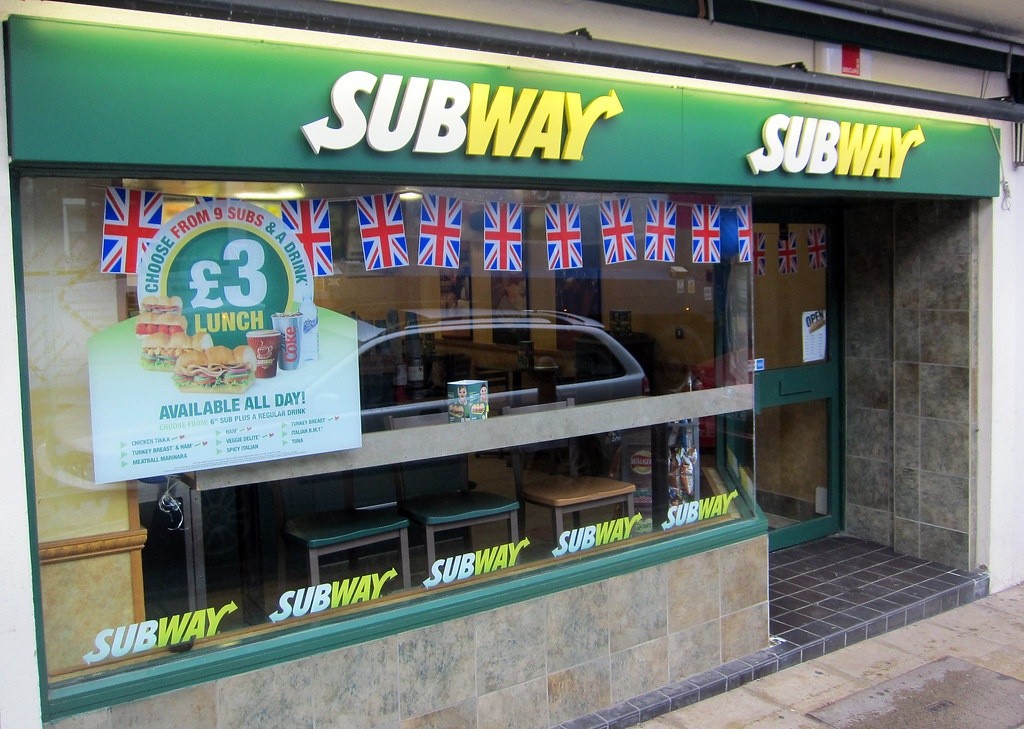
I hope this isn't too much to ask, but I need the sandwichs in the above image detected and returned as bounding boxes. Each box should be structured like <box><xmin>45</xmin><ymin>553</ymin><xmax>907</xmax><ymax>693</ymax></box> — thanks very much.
<box><xmin>134</xmin><ymin>296</ymin><xmax>256</xmax><ymax>393</ymax></box>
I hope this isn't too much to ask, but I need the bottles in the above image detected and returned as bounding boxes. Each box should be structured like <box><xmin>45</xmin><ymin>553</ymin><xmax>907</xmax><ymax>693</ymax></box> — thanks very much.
<box><xmin>298</xmin><ymin>294</ymin><xmax>320</xmax><ymax>363</ymax></box>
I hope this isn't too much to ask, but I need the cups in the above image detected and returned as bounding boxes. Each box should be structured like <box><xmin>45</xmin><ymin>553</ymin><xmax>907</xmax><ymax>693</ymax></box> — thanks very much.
<box><xmin>271</xmin><ymin>313</ymin><xmax>305</xmax><ymax>371</ymax></box>
<box><xmin>246</xmin><ymin>329</ymin><xmax>282</xmax><ymax>378</ymax></box>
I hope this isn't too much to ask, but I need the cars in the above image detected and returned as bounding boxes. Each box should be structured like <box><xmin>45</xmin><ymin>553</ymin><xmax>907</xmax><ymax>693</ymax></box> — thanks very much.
<box><xmin>691</xmin><ymin>345</ymin><xmax>752</xmax><ymax>454</ymax></box>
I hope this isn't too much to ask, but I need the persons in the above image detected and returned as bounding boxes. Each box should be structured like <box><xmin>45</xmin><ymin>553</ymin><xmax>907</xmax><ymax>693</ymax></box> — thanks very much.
<box><xmin>556</xmin><ymin>273</ymin><xmax>593</xmax><ymax>335</ymax></box>
<box><xmin>479</xmin><ymin>384</ymin><xmax>488</xmax><ymax>412</ymax></box>
<box><xmin>457</xmin><ymin>386</ymin><xmax>470</xmax><ymax>418</ymax></box>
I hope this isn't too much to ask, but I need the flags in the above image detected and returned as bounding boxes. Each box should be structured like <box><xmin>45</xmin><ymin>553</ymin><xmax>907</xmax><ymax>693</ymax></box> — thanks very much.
<box><xmin>777</xmin><ymin>232</ymin><xmax>798</xmax><ymax>276</ymax></box>
<box><xmin>545</xmin><ymin>202</ymin><xmax>583</xmax><ymax>270</ymax></box>
<box><xmin>753</xmin><ymin>232</ymin><xmax>766</xmax><ymax>275</ymax></box>
<box><xmin>281</xmin><ymin>197</ymin><xmax>333</xmax><ymax>277</ymax></box>
<box><xmin>691</xmin><ymin>204</ymin><xmax>721</xmax><ymax>263</ymax></box>
<box><xmin>417</xmin><ymin>192</ymin><xmax>463</xmax><ymax>269</ymax></box>
<box><xmin>194</xmin><ymin>196</ymin><xmax>242</xmax><ymax>205</ymax></box>
<box><xmin>644</xmin><ymin>198</ymin><xmax>677</xmax><ymax>263</ymax></box>
<box><xmin>736</xmin><ymin>205</ymin><xmax>752</xmax><ymax>262</ymax></box>
<box><xmin>807</xmin><ymin>228</ymin><xmax>827</xmax><ymax>270</ymax></box>
<box><xmin>99</xmin><ymin>185</ymin><xmax>165</xmax><ymax>273</ymax></box>
<box><xmin>483</xmin><ymin>201</ymin><xmax>523</xmax><ymax>271</ymax></box>
<box><xmin>599</xmin><ymin>198</ymin><xmax>637</xmax><ymax>265</ymax></box>
<box><xmin>356</xmin><ymin>192</ymin><xmax>410</xmax><ymax>272</ymax></box>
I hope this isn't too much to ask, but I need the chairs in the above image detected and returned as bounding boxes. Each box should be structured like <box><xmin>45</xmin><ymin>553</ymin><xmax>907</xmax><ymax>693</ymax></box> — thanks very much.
<box><xmin>501</xmin><ymin>397</ymin><xmax>636</xmax><ymax>553</ymax></box>
<box><xmin>384</xmin><ymin>412</ymin><xmax>520</xmax><ymax>580</ymax></box>
<box><xmin>277</xmin><ymin>461</ymin><xmax>410</xmax><ymax>596</ymax></box>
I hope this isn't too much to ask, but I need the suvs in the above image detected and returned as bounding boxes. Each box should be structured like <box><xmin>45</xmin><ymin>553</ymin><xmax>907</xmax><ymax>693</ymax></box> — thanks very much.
<box><xmin>142</xmin><ymin>309</ymin><xmax>657</xmax><ymax>549</ymax></box>
